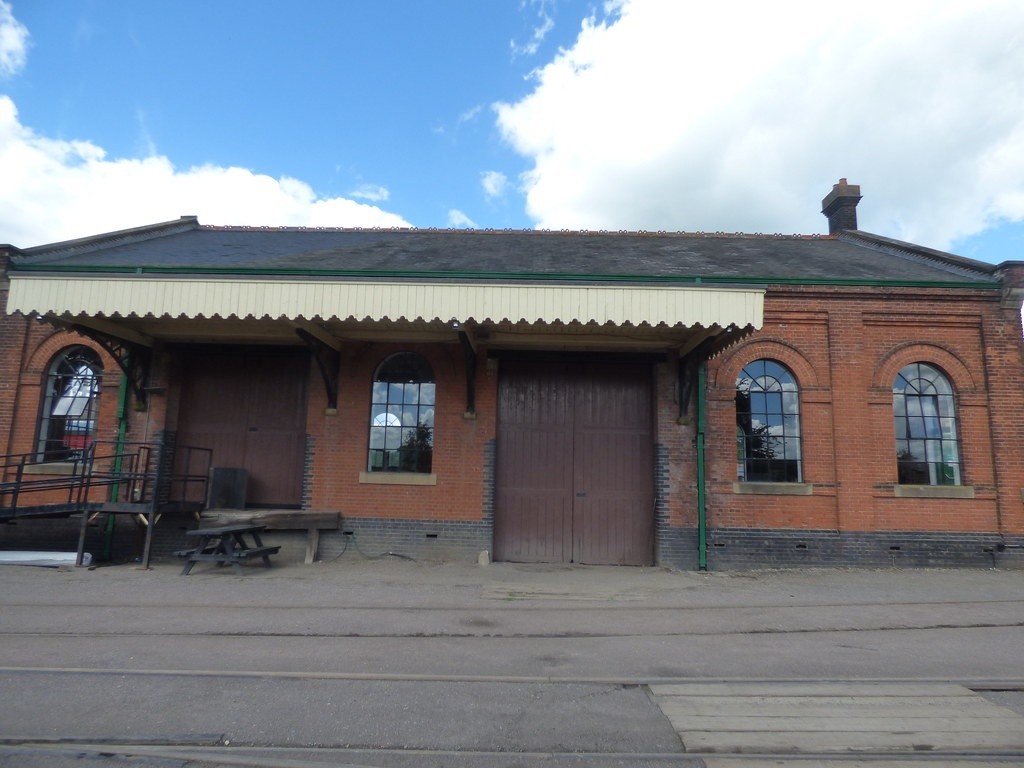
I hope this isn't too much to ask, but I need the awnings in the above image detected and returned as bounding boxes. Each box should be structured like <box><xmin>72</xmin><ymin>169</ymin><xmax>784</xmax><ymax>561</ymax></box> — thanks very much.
<box><xmin>5</xmin><ymin>266</ymin><xmax>764</xmax><ymax>572</ymax></box>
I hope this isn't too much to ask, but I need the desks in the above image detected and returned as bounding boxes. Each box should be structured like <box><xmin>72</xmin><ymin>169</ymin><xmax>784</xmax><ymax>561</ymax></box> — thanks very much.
<box><xmin>175</xmin><ymin>526</ymin><xmax>282</xmax><ymax>577</ymax></box>
